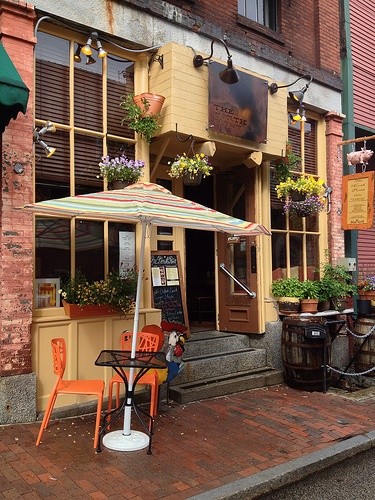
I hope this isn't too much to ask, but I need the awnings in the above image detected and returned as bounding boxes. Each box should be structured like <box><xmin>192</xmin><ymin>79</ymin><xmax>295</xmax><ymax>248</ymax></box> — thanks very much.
<box><xmin>0</xmin><ymin>42</ymin><xmax>30</xmax><ymax>134</ymax></box>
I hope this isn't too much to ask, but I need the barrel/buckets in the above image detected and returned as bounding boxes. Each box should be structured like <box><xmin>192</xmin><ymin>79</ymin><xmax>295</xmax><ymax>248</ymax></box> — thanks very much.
<box><xmin>280</xmin><ymin>317</ymin><xmax>331</xmax><ymax>391</ymax></box>
<box><xmin>353</xmin><ymin>317</ymin><xmax>375</xmax><ymax>378</ymax></box>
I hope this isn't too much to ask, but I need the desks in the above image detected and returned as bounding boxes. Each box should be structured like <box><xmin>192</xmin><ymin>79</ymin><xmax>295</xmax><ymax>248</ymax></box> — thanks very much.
<box><xmin>278</xmin><ymin>306</ymin><xmax>356</xmax><ymax>318</ymax></box>
<box><xmin>94</xmin><ymin>350</ymin><xmax>168</xmax><ymax>455</ymax></box>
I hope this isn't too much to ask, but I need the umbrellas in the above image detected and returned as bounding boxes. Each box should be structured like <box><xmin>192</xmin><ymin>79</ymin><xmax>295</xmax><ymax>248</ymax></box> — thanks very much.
<box><xmin>35</xmin><ymin>187</ymin><xmax>119</xmax><ymax>252</ymax></box>
<box><xmin>13</xmin><ymin>181</ymin><xmax>272</xmax><ymax>435</ymax></box>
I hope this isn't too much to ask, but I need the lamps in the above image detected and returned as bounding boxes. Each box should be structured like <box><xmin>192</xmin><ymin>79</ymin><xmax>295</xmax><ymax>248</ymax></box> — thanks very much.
<box><xmin>270</xmin><ymin>74</ymin><xmax>312</xmax><ymax>107</ymax></box>
<box><xmin>81</xmin><ymin>32</ymin><xmax>108</xmax><ymax>58</ymax></box>
<box><xmin>33</xmin><ymin>121</ymin><xmax>57</xmax><ymax>158</ymax></box>
<box><xmin>194</xmin><ymin>38</ymin><xmax>240</xmax><ymax>84</ymax></box>
<box><xmin>74</xmin><ymin>43</ymin><xmax>96</xmax><ymax>64</ymax></box>
<box><xmin>293</xmin><ymin>106</ymin><xmax>307</xmax><ymax>121</ymax></box>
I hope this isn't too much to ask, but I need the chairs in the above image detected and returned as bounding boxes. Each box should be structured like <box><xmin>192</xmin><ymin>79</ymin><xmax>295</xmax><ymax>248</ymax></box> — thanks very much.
<box><xmin>107</xmin><ymin>332</ymin><xmax>159</xmax><ymax>435</ymax></box>
<box><xmin>36</xmin><ymin>337</ymin><xmax>105</xmax><ymax>449</ymax></box>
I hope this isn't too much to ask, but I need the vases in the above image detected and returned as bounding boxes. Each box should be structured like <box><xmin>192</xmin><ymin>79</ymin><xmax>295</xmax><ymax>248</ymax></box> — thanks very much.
<box><xmin>289</xmin><ymin>189</ymin><xmax>305</xmax><ymax>201</ymax></box>
<box><xmin>62</xmin><ymin>298</ymin><xmax>133</xmax><ymax>318</ymax></box>
<box><xmin>111</xmin><ymin>179</ymin><xmax>133</xmax><ymax>190</ymax></box>
<box><xmin>184</xmin><ymin>168</ymin><xmax>201</xmax><ymax>186</ymax></box>
<box><xmin>359</xmin><ymin>289</ymin><xmax>375</xmax><ymax>300</ymax></box>
<box><xmin>296</xmin><ymin>207</ymin><xmax>310</xmax><ymax>217</ymax></box>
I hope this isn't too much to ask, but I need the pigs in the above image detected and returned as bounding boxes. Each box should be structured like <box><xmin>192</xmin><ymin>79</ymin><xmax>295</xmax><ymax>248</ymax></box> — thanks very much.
<box><xmin>347</xmin><ymin>150</ymin><xmax>374</xmax><ymax>166</ymax></box>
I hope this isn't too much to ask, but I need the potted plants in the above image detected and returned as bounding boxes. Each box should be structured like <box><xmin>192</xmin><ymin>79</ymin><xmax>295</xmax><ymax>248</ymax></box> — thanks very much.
<box><xmin>118</xmin><ymin>93</ymin><xmax>166</xmax><ymax>146</ymax></box>
<box><xmin>270</xmin><ymin>248</ymin><xmax>358</xmax><ymax>313</ymax></box>
<box><xmin>273</xmin><ymin>142</ymin><xmax>303</xmax><ymax>183</ymax></box>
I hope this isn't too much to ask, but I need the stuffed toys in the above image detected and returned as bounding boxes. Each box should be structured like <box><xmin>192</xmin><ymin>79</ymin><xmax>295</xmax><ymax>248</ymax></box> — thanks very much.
<box><xmin>165</xmin><ymin>332</ymin><xmax>185</xmax><ymax>382</ymax></box>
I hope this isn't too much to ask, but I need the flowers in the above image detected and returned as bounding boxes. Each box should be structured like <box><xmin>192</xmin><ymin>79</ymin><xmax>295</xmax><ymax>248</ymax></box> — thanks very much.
<box><xmin>95</xmin><ymin>150</ymin><xmax>145</xmax><ymax>184</ymax></box>
<box><xmin>58</xmin><ymin>261</ymin><xmax>149</xmax><ymax>321</ymax></box>
<box><xmin>362</xmin><ymin>275</ymin><xmax>375</xmax><ymax>291</ymax></box>
<box><xmin>274</xmin><ymin>175</ymin><xmax>324</xmax><ymax>222</ymax></box>
<box><xmin>166</xmin><ymin>152</ymin><xmax>213</xmax><ymax>179</ymax></box>
